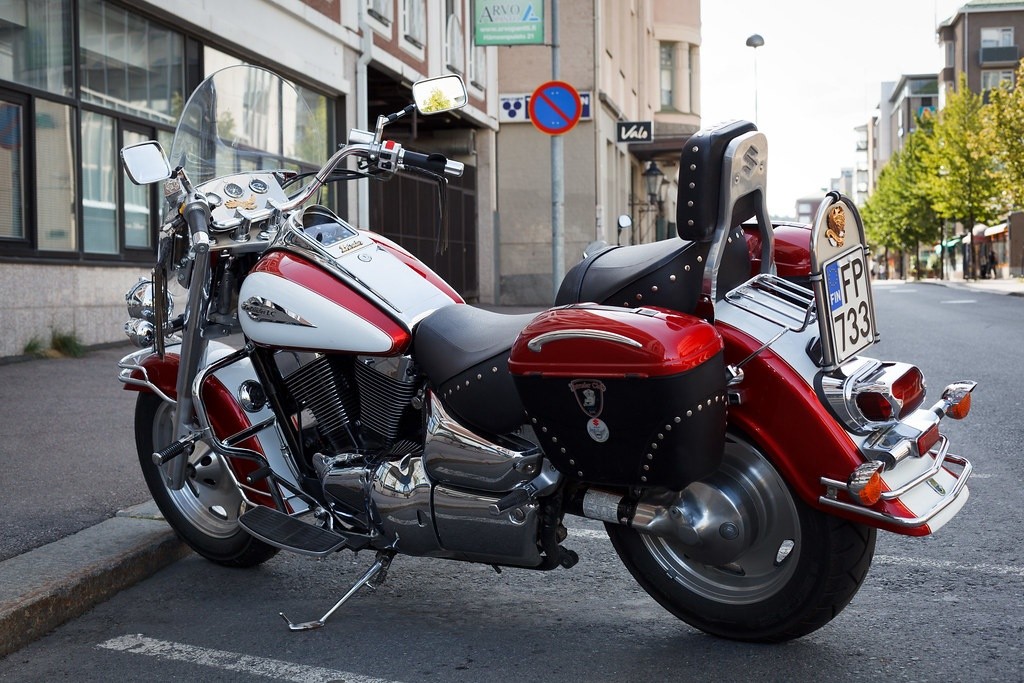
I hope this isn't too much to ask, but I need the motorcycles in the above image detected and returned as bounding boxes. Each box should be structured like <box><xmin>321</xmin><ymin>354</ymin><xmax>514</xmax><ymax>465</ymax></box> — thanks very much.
<box><xmin>116</xmin><ymin>64</ymin><xmax>980</xmax><ymax>645</ymax></box>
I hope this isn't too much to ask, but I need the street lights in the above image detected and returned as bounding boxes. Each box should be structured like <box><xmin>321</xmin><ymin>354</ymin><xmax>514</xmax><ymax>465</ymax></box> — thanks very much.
<box><xmin>746</xmin><ymin>33</ymin><xmax>765</xmax><ymax>126</ymax></box>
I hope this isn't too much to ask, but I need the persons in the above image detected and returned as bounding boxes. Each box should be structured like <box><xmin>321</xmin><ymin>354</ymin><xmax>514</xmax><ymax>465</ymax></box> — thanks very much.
<box><xmin>871</xmin><ymin>258</ymin><xmax>886</xmax><ymax>280</ymax></box>
<box><xmin>978</xmin><ymin>245</ymin><xmax>998</xmax><ymax>279</ymax></box>
